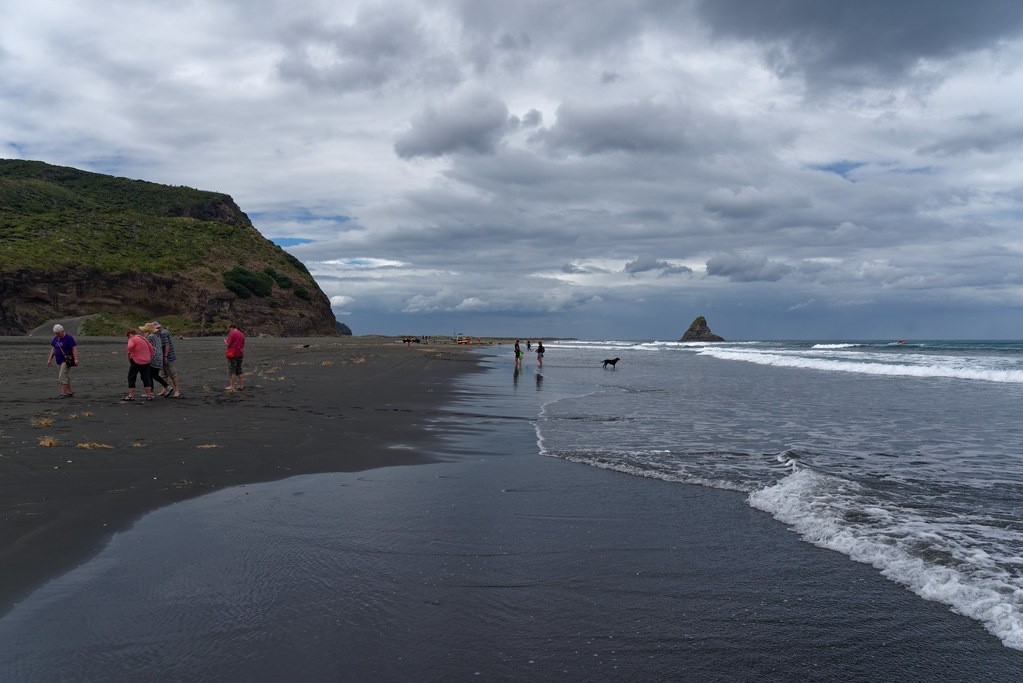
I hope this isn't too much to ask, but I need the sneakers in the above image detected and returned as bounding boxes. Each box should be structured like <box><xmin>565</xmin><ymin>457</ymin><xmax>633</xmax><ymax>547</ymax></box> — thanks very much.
<box><xmin>164</xmin><ymin>387</ymin><xmax>173</xmax><ymax>397</ymax></box>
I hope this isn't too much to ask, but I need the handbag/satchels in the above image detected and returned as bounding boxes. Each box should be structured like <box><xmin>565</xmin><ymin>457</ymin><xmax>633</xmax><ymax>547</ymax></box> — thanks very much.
<box><xmin>65</xmin><ymin>354</ymin><xmax>78</xmax><ymax>367</ymax></box>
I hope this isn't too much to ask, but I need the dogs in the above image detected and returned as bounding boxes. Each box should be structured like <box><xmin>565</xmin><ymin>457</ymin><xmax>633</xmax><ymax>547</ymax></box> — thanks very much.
<box><xmin>600</xmin><ymin>358</ymin><xmax>620</xmax><ymax>369</ymax></box>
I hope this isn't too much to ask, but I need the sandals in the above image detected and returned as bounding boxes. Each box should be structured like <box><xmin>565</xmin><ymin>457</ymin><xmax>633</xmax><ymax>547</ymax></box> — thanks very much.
<box><xmin>120</xmin><ymin>396</ymin><xmax>135</xmax><ymax>401</ymax></box>
<box><xmin>146</xmin><ymin>396</ymin><xmax>152</xmax><ymax>401</ymax></box>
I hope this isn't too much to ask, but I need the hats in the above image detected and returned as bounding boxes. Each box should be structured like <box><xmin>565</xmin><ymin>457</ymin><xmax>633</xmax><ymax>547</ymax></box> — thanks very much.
<box><xmin>139</xmin><ymin>323</ymin><xmax>159</xmax><ymax>333</ymax></box>
<box><xmin>151</xmin><ymin>322</ymin><xmax>162</xmax><ymax>328</ymax></box>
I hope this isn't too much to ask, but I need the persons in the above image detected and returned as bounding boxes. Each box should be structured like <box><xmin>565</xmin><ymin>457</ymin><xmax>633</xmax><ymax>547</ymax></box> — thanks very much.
<box><xmin>408</xmin><ymin>338</ymin><xmax>414</xmax><ymax>351</ymax></box>
<box><xmin>526</xmin><ymin>340</ymin><xmax>532</xmax><ymax>351</ymax></box>
<box><xmin>137</xmin><ymin>322</ymin><xmax>174</xmax><ymax>398</ymax></box>
<box><xmin>514</xmin><ymin>340</ymin><xmax>521</xmax><ymax>364</ymax></box>
<box><xmin>152</xmin><ymin>321</ymin><xmax>181</xmax><ymax>398</ymax></box>
<box><xmin>535</xmin><ymin>340</ymin><xmax>545</xmax><ymax>368</ymax></box>
<box><xmin>46</xmin><ymin>324</ymin><xmax>79</xmax><ymax>399</ymax></box>
<box><xmin>519</xmin><ymin>351</ymin><xmax>523</xmax><ymax>364</ymax></box>
<box><xmin>223</xmin><ymin>323</ymin><xmax>245</xmax><ymax>390</ymax></box>
<box><xmin>121</xmin><ymin>327</ymin><xmax>155</xmax><ymax>401</ymax></box>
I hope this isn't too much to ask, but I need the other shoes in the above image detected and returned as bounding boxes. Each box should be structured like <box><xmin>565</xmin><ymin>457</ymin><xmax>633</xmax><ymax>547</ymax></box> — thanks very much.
<box><xmin>56</xmin><ymin>393</ymin><xmax>74</xmax><ymax>399</ymax></box>
